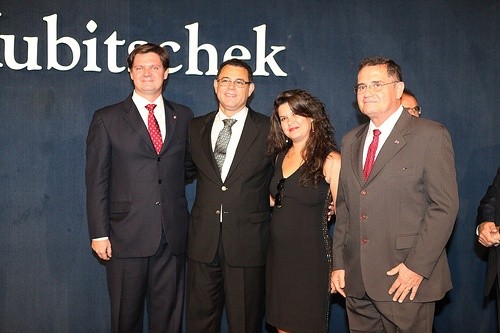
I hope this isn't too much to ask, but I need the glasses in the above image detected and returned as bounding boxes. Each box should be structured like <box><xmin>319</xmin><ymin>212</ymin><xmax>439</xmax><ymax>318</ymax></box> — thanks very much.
<box><xmin>274</xmin><ymin>177</ymin><xmax>286</xmax><ymax>208</ymax></box>
<box><xmin>404</xmin><ymin>105</ymin><xmax>422</xmax><ymax>115</ymax></box>
<box><xmin>353</xmin><ymin>80</ymin><xmax>398</xmax><ymax>94</ymax></box>
<box><xmin>216</xmin><ymin>77</ymin><xmax>252</xmax><ymax>87</ymax></box>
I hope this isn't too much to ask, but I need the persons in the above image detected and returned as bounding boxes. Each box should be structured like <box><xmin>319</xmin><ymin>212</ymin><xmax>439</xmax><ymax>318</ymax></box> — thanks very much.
<box><xmin>182</xmin><ymin>59</ymin><xmax>334</xmax><ymax>333</ymax></box>
<box><xmin>401</xmin><ymin>90</ymin><xmax>421</xmax><ymax>118</ymax></box>
<box><xmin>330</xmin><ymin>56</ymin><xmax>460</xmax><ymax>333</ymax></box>
<box><xmin>475</xmin><ymin>165</ymin><xmax>500</xmax><ymax>322</ymax></box>
<box><xmin>84</xmin><ymin>42</ymin><xmax>195</xmax><ymax>333</ymax></box>
<box><xmin>263</xmin><ymin>90</ymin><xmax>341</xmax><ymax>333</ymax></box>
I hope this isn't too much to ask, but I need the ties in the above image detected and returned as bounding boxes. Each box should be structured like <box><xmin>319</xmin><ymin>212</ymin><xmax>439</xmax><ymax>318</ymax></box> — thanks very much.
<box><xmin>145</xmin><ymin>104</ymin><xmax>163</xmax><ymax>154</ymax></box>
<box><xmin>213</xmin><ymin>119</ymin><xmax>238</xmax><ymax>177</ymax></box>
<box><xmin>362</xmin><ymin>127</ymin><xmax>383</xmax><ymax>184</ymax></box>
